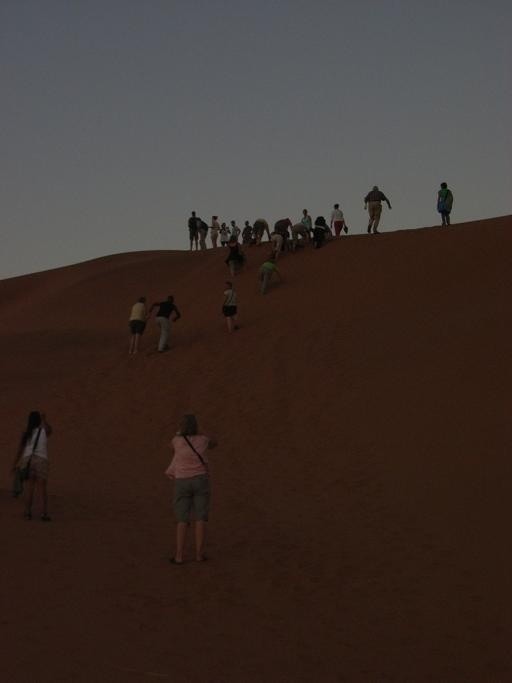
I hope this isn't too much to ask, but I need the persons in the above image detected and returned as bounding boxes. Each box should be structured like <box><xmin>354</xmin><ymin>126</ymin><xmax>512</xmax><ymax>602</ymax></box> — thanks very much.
<box><xmin>363</xmin><ymin>186</ymin><xmax>391</xmax><ymax>235</ymax></box>
<box><xmin>166</xmin><ymin>409</ymin><xmax>218</xmax><ymax>565</ymax></box>
<box><xmin>437</xmin><ymin>182</ymin><xmax>454</xmax><ymax>228</ymax></box>
<box><xmin>225</xmin><ymin>235</ymin><xmax>244</xmax><ymax>275</ymax></box>
<box><xmin>258</xmin><ymin>256</ymin><xmax>281</xmax><ymax>294</ymax></box>
<box><xmin>222</xmin><ymin>281</ymin><xmax>238</xmax><ymax>334</ymax></box>
<box><xmin>6</xmin><ymin>409</ymin><xmax>52</xmax><ymax>522</ymax></box>
<box><xmin>128</xmin><ymin>295</ymin><xmax>180</xmax><ymax>352</ymax></box>
<box><xmin>188</xmin><ymin>204</ymin><xmax>346</xmax><ymax>260</ymax></box>
